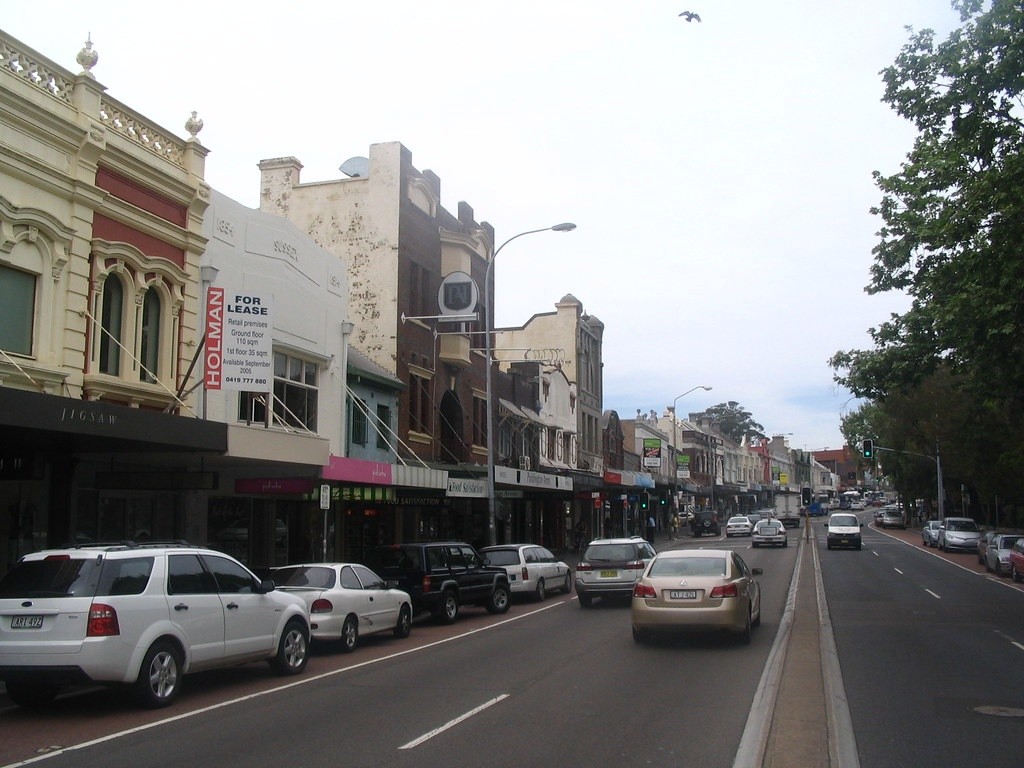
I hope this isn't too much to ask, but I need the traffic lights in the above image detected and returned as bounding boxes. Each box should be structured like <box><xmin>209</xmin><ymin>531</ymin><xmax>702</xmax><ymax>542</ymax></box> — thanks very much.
<box><xmin>640</xmin><ymin>492</ymin><xmax>649</xmax><ymax>512</ymax></box>
<box><xmin>802</xmin><ymin>488</ymin><xmax>811</xmax><ymax>506</ymax></box>
<box><xmin>659</xmin><ymin>491</ymin><xmax>667</xmax><ymax>507</ymax></box>
<box><xmin>862</xmin><ymin>440</ymin><xmax>873</xmax><ymax>458</ymax></box>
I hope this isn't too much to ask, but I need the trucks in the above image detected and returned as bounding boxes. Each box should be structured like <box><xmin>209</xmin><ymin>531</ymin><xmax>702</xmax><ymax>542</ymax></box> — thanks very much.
<box><xmin>773</xmin><ymin>492</ymin><xmax>801</xmax><ymax>528</ymax></box>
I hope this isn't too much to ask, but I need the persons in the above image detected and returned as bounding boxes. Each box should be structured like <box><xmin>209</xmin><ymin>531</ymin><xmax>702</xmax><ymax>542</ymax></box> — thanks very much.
<box><xmin>575</xmin><ymin>516</ymin><xmax>589</xmax><ymax>551</ymax></box>
<box><xmin>672</xmin><ymin>513</ymin><xmax>680</xmax><ymax>540</ymax></box>
<box><xmin>917</xmin><ymin>507</ymin><xmax>922</xmax><ymax>522</ymax></box>
<box><xmin>647</xmin><ymin>513</ymin><xmax>656</xmax><ymax>547</ymax></box>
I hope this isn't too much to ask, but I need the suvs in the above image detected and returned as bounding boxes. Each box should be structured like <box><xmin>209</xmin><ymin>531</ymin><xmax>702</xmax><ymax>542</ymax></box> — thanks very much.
<box><xmin>364</xmin><ymin>541</ymin><xmax>513</xmax><ymax>624</ymax></box>
<box><xmin>574</xmin><ymin>535</ymin><xmax>658</xmax><ymax>607</ymax></box>
<box><xmin>0</xmin><ymin>540</ymin><xmax>313</xmax><ymax>711</ymax></box>
<box><xmin>694</xmin><ymin>511</ymin><xmax>722</xmax><ymax>537</ymax></box>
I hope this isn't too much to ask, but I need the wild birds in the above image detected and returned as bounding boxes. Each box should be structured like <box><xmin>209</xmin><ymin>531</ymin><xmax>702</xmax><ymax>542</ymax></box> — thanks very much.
<box><xmin>679</xmin><ymin>11</ymin><xmax>702</xmax><ymax>22</ymax></box>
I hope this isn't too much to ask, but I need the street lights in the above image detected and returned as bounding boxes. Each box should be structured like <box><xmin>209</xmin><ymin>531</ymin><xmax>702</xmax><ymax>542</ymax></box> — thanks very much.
<box><xmin>673</xmin><ymin>385</ymin><xmax>712</xmax><ymax>517</ymax></box>
<box><xmin>485</xmin><ymin>222</ymin><xmax>577</xmax><ymax>544</ymax></box>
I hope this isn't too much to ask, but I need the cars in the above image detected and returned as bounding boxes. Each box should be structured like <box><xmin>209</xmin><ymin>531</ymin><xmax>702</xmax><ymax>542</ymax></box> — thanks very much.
<box><xmin>751</xmin><ymin>519</ymin><xmax>787</xmax><ymax>547</ymax></box>
<box><xmin>269</xmin><ymin>563</ymin><xmax>415</xmax><ymax>654</ymax></box>
<box><xmin>801</xmin><ymin>490</ymin><xmax>924</xmax><ymax>529</ymax></box>
<box><xmin>824</xmin><ymin>513</ymin><xmax>864</xmax><ymax>551</ymax></box>
<box><xmin>676</xmin><ymin>512</ymin><xmax>693</xmax><ymax>527</ymax></box>
<box><xmin>922</xmin><ymin>517</ymin><xmax>1024</xmax><ymax>583</ymax></box>
<box><xmin>482</xmin><ymin>543</ymin><xmax>573</xmax><ymax>603</ymax></box>
<box><xmin>726</xmin><ymin>509</ymin><xmax>775</xmax><ymax>538</ymax></box>
<box><xmin>630</xmin><ymin>549</ymin><xmax>764</xmax><ymax>645</ymax></box>
